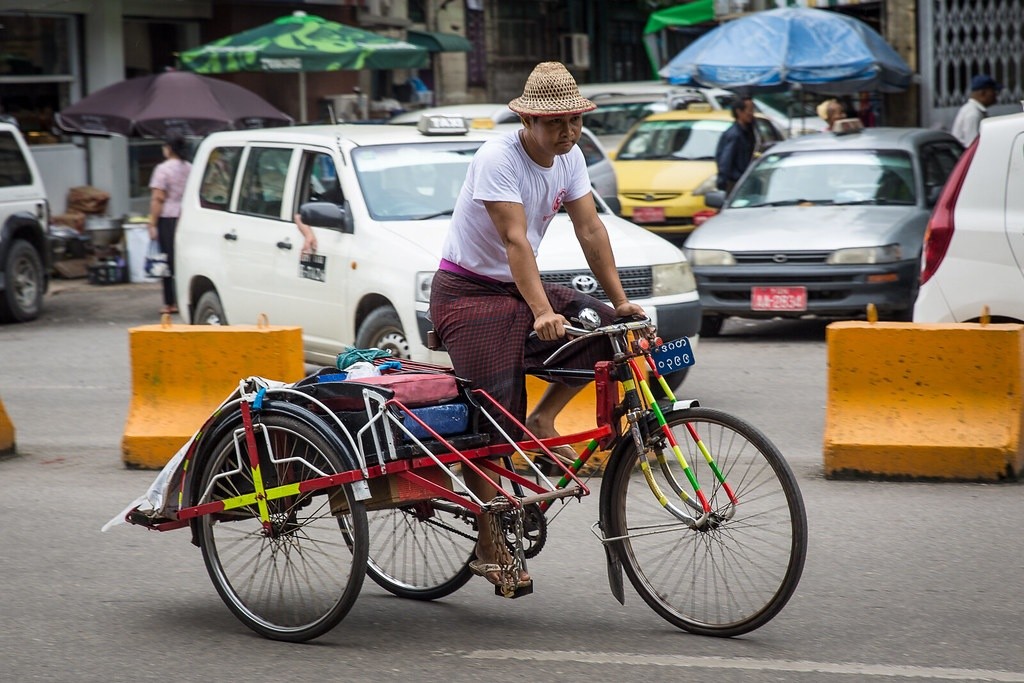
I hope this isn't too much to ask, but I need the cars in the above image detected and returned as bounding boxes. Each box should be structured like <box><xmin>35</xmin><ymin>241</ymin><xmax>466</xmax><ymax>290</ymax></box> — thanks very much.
<box><xmin>673</xmin><ymin>122</ymin><xmax>973</xmax><ymax>339</ymax></box>
<box><xmin>0</xmin><ymin>117</ymin><xmax>55</xmax><ymax>322</ymax></box>
<box><xmin>386</xmin><ymin>84</ymin><xmax>699</xmax><ymax>218</ymax></box>
<box><xmin>910</xmin><ymin>104</ymin><xmax>1024</xmax><ymax>335</ymax></box>
<box><xmin>610</xmin><ymin>101</ymin><xmax>786</xmax><ymax>248</ymax></box>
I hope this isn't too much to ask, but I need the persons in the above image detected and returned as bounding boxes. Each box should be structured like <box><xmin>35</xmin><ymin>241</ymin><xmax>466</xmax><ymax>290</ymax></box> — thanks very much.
<box><xmin>817</xmin><ymin>99</ymin><xmax>846</xmax><ymax>132</ymax></box>
<box><xmin>716</xmin><ymin>94</ymin><xmax>764</xmax><ymax>201</ymax></box>
<box><xmin>148</xmin><ymin>135</ymin><xmax>194</xmax><ymax>314</ymax></box>
<box><xmin>293</xmin><ymin>205</ymin><xmax>345</xmax><ymax>254</ymax></box>
<box><xmin>951</xmin><ymin>74</ymin><xmax>1008</xmax><ymax>149</ymax></box>
<box><xmin>429</xmin><ymin>61</ymin><xmax>648</xmax><ymax>585</ymax></box>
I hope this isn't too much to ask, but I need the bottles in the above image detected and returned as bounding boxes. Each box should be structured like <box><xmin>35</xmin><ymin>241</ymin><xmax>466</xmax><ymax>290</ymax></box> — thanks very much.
<box><xmin>97</xmin><ymin>257</ymin><xmax>117</xmax><ymax>280</ymax></box>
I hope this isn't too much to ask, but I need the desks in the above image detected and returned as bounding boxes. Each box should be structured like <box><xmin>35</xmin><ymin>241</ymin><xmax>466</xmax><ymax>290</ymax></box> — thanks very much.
<box><xmin>87</xmin><ymin>226</ymin><xmax>123</xmax><ymax>244</ymax></box>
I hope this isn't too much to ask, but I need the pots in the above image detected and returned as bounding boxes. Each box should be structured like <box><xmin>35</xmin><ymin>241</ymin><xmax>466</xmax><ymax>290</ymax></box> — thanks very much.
<box><xmin>85</xmin><ymin>218</ymin><xmax>123</xmax><ymax>244</ymax></box>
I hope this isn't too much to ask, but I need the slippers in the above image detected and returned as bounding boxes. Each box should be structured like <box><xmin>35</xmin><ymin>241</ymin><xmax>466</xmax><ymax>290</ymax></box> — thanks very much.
<box><xmin>159</xmin><ymin>306</ymin><xmax>179</xmax><ymax>313</ymax></box>
<box><xmin>469</xmin><ymin>558</ymin><xmax>531</xmax><ymax>587</ymax></box>
<box><xmin>525</xmin><ymin>445</ymin><xmax>589</xmax><ymax>468</ymax></box>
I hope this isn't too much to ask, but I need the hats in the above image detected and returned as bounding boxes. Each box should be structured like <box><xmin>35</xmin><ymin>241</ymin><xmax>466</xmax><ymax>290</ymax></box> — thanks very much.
<box><xmin>508</xmin><ymin>62</ymin><xmax>597</xmax><ymax>116</ymax></box>
<box><xmin>972</xmin><ymin>76</ymin><xmax>1004</xmax><ymax>90</ymax></box>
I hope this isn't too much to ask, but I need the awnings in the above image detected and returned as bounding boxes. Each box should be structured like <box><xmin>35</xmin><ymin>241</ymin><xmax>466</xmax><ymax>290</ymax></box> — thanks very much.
<box><xmin>406</xmin><ymin>29</ymin><xmax>472</xmax><ymax>52</ymax></box>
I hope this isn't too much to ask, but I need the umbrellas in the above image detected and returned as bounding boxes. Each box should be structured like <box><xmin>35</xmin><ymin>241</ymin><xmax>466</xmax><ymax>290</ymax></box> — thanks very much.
<box><xmin>50</xmin><ymin>68</ymin><xmax>294</xmax><ymax>141</ymax></box>
<box><xmin>657</xmin><ymin>4</ymin><xmax>914</xmax><ymax>90</ymax></box>
<box><xmin>173</xmin><ymin>11</ymin><xmax>431</xmax><ymax>124</ymax></box>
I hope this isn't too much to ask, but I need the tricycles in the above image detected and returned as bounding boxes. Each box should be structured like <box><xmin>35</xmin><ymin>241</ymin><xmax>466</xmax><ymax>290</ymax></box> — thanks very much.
<box><xmin>122</xmin><ymin>308</ymin><xmax>810</xmax><ymax>648</ymax></box>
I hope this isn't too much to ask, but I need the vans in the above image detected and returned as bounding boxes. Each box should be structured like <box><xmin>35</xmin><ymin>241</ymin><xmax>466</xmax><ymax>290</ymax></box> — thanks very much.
<box><xmin>169</xmin><ymin>115</ymin><xmax>703</xmax><ymax>396</ymax></box>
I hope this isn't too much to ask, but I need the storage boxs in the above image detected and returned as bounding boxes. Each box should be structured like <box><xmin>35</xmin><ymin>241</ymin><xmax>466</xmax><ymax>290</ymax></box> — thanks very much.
<box><xmin>48</xmin><ymin>236</ymin><xmax>81</xmax><ymax>262</ymax></box>
<box><xmin>84</xmin><ymin>262</ymin><xmax>128</xmax><ymax>286</ymax></box>
<box><xmin>66</xmin><ymin>185</ymin><xmax>109</xmax><ymax>212</ymax></box>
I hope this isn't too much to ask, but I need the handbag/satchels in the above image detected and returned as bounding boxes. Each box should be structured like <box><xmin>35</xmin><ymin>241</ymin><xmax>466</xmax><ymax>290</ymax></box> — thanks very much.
<box><xmin>146</xmin><ymin>241</ymin><xmax>172</xmax><ymax>278</ymax></box>
